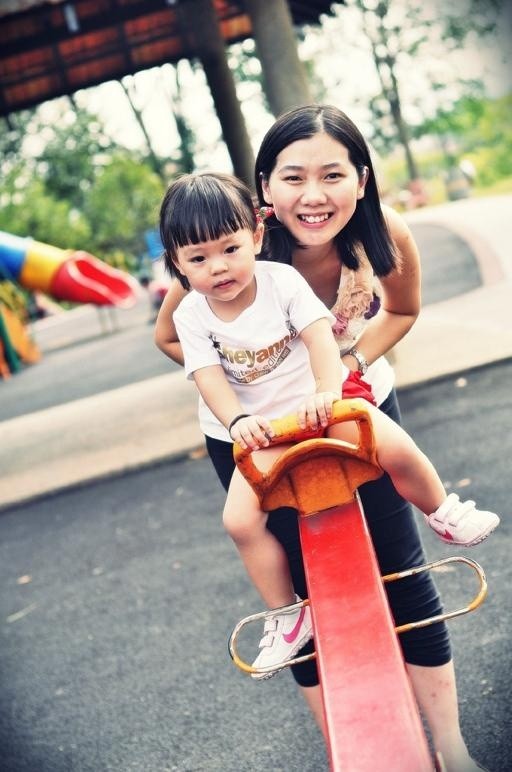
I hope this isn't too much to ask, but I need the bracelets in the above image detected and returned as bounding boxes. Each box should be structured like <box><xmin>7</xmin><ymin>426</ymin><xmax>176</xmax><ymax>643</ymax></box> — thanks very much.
<box><xmin>229</xmin><ymin>414</ymin><xmax>250</xmax><ymax>432</ymax></box>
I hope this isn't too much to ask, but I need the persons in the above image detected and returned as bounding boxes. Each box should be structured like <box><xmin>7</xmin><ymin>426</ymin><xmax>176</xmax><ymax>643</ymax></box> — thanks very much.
<box><xmin>154</xmin><ymin>106</ymin><xmax>486</xmax><ymax>772</ymax></box>
<box><xmin>406</xmin><ymin>170</ymin><xmax>424</xmax><ymax>209</ymax></box>
<box><xmin>159</xmin><ymin>173</ymin><xmax>500</xmax><ymax>681</ymax></box>
<box><xmin>444</xmin><ymin>162</ymin><xmax>470</xmax><ymax>198</ymax></box>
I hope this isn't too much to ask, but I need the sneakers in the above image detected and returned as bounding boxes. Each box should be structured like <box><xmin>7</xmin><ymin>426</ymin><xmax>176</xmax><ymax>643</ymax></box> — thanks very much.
<box><xmin>423</xmin><ymin>493</ymin><xmax>501</xmax><ymax>548</ymax></box>
<box><xmin>250</xmin><ymin>594</ymin><xmax>313</xmax><ymax>682</ymax></box>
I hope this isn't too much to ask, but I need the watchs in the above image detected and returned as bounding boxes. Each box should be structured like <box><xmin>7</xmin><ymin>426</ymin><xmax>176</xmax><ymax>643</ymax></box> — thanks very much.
<box><xmin>340</xmin><ymin>350</ymin><xmax>368</xmax><ymax>378</ymax></box>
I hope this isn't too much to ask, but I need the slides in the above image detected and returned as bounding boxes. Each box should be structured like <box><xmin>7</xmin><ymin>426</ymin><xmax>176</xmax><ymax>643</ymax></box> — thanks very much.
<box><xmin>0</xmin><ymin>231</ymin><xmax>141</xmax><ymax>306</ymax></box>
